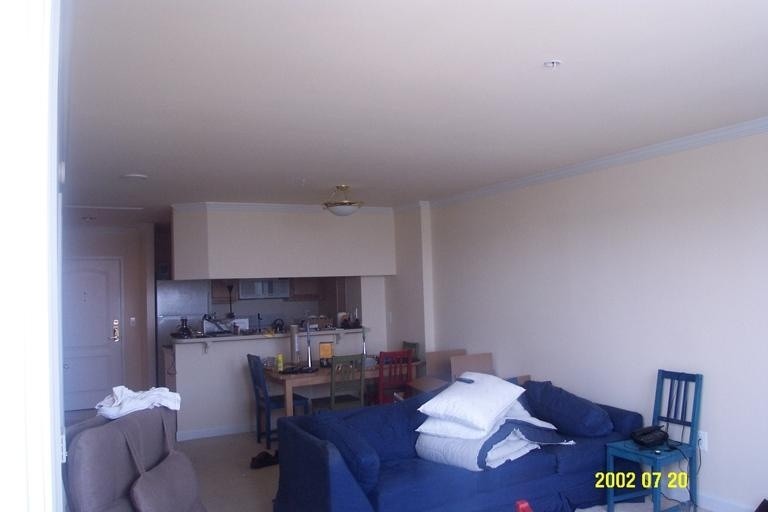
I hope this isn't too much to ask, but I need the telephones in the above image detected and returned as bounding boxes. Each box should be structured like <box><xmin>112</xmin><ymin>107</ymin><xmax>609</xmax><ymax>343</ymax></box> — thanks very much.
<box><xmin>630</xmin><ymin>424</ymin><xmax>668</xmax><ymax>447</ymax></box>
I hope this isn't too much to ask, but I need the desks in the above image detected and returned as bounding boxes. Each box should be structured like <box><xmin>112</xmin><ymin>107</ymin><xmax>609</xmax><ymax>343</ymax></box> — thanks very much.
<box><xmin>266</xmin><ymin>354</ymin><xmax>424</xmax><ymax>416</ymax></box>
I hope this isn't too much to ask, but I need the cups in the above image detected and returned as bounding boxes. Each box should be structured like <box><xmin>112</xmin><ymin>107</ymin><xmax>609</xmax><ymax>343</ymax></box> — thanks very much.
<box><xmin>267</xmin><ymin>357</ymin><xmax>275</xmax><ymax>366</ymax></box>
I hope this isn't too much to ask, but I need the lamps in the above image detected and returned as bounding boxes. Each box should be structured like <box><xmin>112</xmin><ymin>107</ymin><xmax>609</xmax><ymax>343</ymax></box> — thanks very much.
<box><xmin>321</xmin><ymin>184</ymin><xmax>364</xmax><ymax>216</ymax></box>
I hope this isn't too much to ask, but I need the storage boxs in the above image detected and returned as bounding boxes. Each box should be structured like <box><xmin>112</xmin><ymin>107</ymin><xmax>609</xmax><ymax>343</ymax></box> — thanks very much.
<box><xmin>407</xmin><ymin>350</ymin><xmax>531</xmax><ymax>395</ymax></box>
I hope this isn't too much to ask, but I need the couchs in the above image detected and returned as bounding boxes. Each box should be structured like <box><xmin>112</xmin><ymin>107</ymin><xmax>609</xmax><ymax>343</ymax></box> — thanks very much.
<box><xmin>272</xmin><ymin>380</ymin><xmax>647</xmax><ymax>512</ymax></box>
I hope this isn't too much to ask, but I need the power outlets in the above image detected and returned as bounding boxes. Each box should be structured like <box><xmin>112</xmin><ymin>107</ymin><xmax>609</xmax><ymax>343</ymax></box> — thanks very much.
<box><xmin>695</xmin><ymin>431</ymin><xmax>707</xmax><ymax>451</ymax></box>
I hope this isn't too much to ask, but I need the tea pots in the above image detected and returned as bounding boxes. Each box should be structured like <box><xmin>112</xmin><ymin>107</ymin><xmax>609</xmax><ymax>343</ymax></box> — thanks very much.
<box><xmin>272</xmin><ymin>319</ymin><xmax>287</xmax><ymax>334</ymax></box>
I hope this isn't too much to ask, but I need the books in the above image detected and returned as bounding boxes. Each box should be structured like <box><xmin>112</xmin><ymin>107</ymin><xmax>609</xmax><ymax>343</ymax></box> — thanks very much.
<box><xmin>279</xmin><ymin>367</ymin><xmax>319</xmax><ymax>373</ymax></box>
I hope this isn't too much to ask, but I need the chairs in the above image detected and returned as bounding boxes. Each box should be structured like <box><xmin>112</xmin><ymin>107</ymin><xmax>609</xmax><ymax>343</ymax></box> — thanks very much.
<box><xmin>402</xmin><ymin>340</ymin><xmax>420</xmax><ymax>360</ymax></box>
<box><xmin>606</xmin><ymin>368</ymin><xmax>703</xmax><ymax>512</ymax></box>
<box><xmin>311</xmin><ymin>352</ymin><xmax>365</xmax><ymax>414</ymax></box>
<box><xmin>247</xmin><ymin>354</ymin><xmax>310</xmax><ymax>450</ymax></box>
<box><xmin>366</xmin><ymin>349</ymin><xmax>412</xmax><ymax>405</ymax></box>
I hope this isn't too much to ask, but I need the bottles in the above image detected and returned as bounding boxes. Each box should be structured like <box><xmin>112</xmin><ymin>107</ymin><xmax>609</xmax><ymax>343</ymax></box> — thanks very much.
<box><xmin>179</xmin><ymin>319</ymin><xmax>189</xmax><ymax>338</ymax></box>
<box><xmin>277</xmin><ymin>353</ymin><xmax>283</xmax><ymax>372</ymax></box>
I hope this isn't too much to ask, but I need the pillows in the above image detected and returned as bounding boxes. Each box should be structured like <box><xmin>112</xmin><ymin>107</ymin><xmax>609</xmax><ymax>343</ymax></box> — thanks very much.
<box><xmin>414</xmin><ymin>400</ymin><xmax>516</xmax><ymax>440</ymax></box>
<box><xmin>417</xmin><ymin>372</ymin><xmax>527</xmax><ymax>431</ymax></box>
